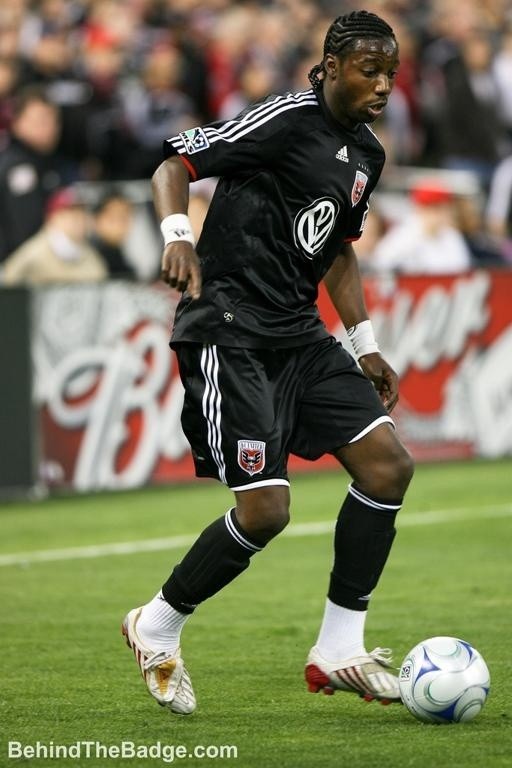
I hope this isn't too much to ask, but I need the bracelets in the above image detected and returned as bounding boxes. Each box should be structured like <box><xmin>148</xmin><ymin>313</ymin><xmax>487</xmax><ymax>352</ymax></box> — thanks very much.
<box><xmin>160</xmin><ymin>214</ymin><xmax>196</xmax><ymax>250</ymax></box>
<box><xmin>346</xmin><ymin>320</ymin><xmax>382</xmax><ymax>361</ymax></box>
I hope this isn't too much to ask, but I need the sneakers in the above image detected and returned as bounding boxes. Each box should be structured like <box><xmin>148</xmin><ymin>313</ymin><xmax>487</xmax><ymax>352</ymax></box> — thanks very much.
<box><xmin>305</xmin><ymin>645</ymin><xmax>400</xmax><ymax>704</ymax></box>
<box><xmin>122</xmin><ymin>606</ymin><xmax>197</xmax><ymax>715</ymax></box>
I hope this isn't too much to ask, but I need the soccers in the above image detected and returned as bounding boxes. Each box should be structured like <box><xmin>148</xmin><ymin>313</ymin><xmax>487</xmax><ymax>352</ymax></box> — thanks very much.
<box><xmin>398</xmin><ymin>636</ymin><xmax>491</xmax><ymax>722</ymax></box>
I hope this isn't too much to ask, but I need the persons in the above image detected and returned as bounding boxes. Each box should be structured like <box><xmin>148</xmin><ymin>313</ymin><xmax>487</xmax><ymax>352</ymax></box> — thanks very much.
<box><xmin>0</xmin><ymin>1</ymin><xmax>512</xmax><ymax>286</ymax></box>
<box><xmin>120</xmin><ymin>10</ymin><xmax>415</xmax><ymax>715</ymax></box>
